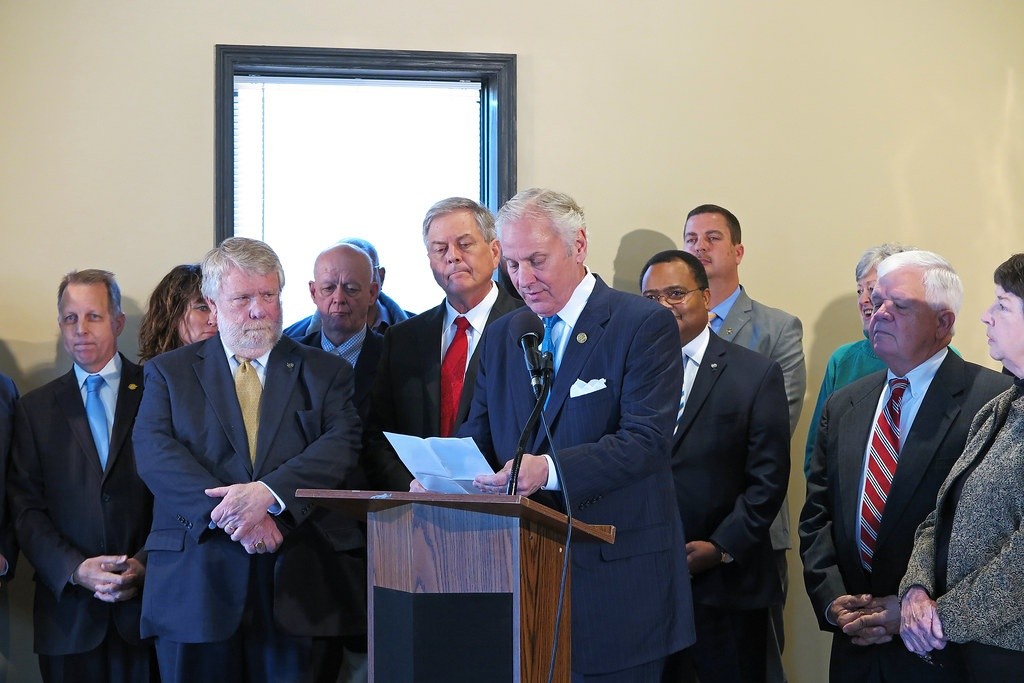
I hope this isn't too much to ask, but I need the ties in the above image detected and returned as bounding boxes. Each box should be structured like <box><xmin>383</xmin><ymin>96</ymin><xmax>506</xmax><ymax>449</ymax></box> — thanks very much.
<box><xmin>707</xmin><ymin>311</ymin><xmax>717</xmax><ymax>328</ymax></box>
<box><xmin>330</xmin><ymin>349</ymin><xmax>340</xmax><ymax>356</ymax></box>
<box><xmin>440</xmin><ymin>316</ymin><xmax>470</xmax><ymax>437</ymax></box>
<box><xmin>541</xmin><ymin>313</ymin><xmax>561</xmax><ymax>413</ymax></box>
<box><xmin>234</xmin><ymin>353</ymin><xmax>263</xmax><ymax>469</ymax></box>
<box><xmin>861</xmin><ymin>376</ymin><xmax>910</xmax><ymax>573</ymax></box>
<box><xmin>85</xmin><ymin>375</ymin><xmax>109</xmax><ymax>473</ymax></box>
<box><xmin>673</xmin><ymin>354</ymin><xmax>688</xmax><ymax>435</ymax></box>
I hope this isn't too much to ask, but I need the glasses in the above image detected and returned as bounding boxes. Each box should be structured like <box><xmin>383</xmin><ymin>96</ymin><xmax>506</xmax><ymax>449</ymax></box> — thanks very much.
<box><xmin>646</xmin><ymin>286</ymin><xmax>705</xmax><ymax>305</ymax></box>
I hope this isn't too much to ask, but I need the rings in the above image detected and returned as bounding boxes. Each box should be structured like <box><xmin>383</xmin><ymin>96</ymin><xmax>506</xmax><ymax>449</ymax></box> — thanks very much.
<box><xmin>226</xmin><ymin>524</ymin><xmax>235</xmax><ymax>531</ymax></box>
<box><xmin>254</xmin><ymin>540</ymin><xmax>263</xmax><ymax>549</ymax></box>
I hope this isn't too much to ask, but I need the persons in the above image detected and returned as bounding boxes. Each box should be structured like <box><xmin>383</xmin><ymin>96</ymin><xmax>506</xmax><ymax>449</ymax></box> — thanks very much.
<box><xmin>288</xmin><ymin>243</ymin><xmax>386</xmax><ymax>683</ymax></box>
<box><xmin>0</xmin><ymin>371</ymin><xmax>23</xmax><ymax>683</ymax></box>
<box><xmin>283</xmin><ymin>238</ymin><xmax>419</xmax><ymax>338</ymax></box>
<box><xmin>355</xmin><ymin>197</ymin><xmax>526</xmax><ymax>490</ymax></box>
<box><xmin>640</xmin><ymin>250</ymin><xmax>791</xmax><ymax>683</ymax></box>
<box><xmin>683</xmin><ymin>203</ymin><xmax>807</xmax><ymax>683</ymax></box>
<box><xmin>803</xmin><ymin>237</ymin><xmax>967</xmax><ymax>478</ymax></box>
<box><xmin>6</xmin><ymin>268</ymin><xmax>162</xmax><ymax>683</ymax></box>
<box><xmin>131</xmin><ymin>236</ymin><xmax>363</xmax><ymax>683</ymax></box>
<box><xmin>798</xmin><ymin>249</ymin><xmax>1023</xmax><ymax>683</ymax></box>
<box><xmin>134</xmin><ymin>263</ymin><xmax>219</xmax><ymax>366</ymax></box>
<box><xmin>409</xmin><ymin>187</ymin><xmax>696</xmax><ymax>683</ymax></box>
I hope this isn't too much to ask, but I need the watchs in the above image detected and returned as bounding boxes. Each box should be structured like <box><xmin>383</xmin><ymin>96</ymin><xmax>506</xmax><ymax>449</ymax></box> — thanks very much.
<box><xmin>710</xmin><ymin>541</ymin><xmax>734</xmax><ymax>563</ymax></box>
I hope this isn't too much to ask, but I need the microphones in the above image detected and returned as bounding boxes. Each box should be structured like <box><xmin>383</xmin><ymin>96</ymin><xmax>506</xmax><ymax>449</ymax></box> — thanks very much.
<box><xmin>508</xmin><ymin>310</ymin><xmax>545</xmax><ymax>402</ymax></box>
